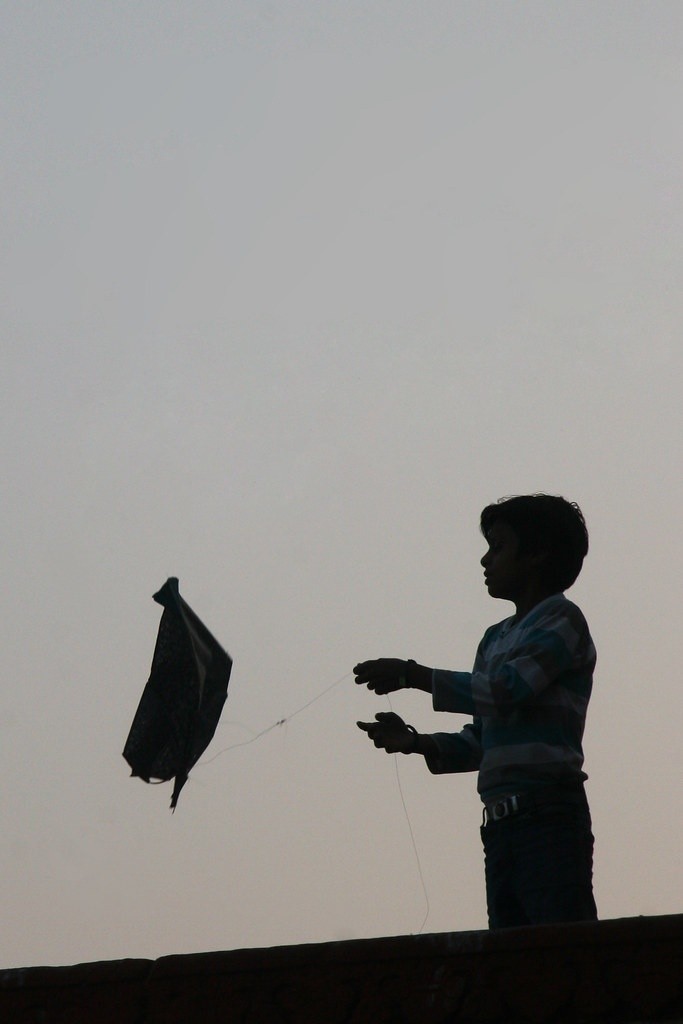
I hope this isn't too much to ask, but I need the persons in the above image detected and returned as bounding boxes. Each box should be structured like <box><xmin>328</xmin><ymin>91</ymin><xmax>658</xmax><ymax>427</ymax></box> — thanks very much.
<box><xmin>352</xmin><ymin>493</ymin><xmax>598</xmax><ymax>931</ymax></box>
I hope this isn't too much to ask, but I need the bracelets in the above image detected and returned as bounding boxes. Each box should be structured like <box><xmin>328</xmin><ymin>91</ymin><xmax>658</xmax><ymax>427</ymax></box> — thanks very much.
<box><xmin>406</xmin><ymin>658</ymin><xmax>417</xmax><ymax>688</ymax></box>
<box><xmin>401</xmin><ymin>725</ymin><xmax>418</xmax><ymax>755</ymax></box>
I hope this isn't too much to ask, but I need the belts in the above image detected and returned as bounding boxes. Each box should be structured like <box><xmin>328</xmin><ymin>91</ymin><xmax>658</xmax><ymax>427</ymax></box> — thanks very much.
<box><xmin>486</xmin><ymin>782</ymin><xmax>563</xmax><ymax>820</ymax></box>
